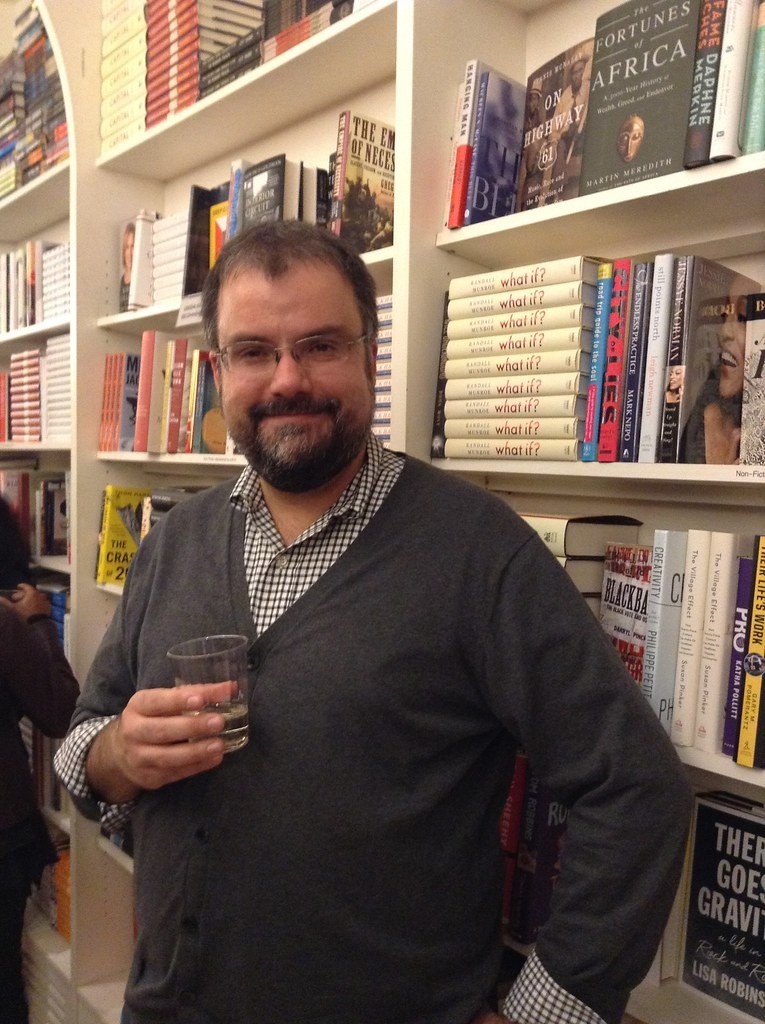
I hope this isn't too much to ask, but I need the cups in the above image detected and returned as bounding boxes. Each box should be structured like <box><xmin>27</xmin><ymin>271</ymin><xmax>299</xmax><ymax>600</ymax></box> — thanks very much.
<box><xmin>0</xmin><ymin>590</ymin><xmax>24</xmax><ymax>603</ymax></box>
<box><xmin>167</xmin><ymin>635</ymin><xmax>249</xmax><ymax>754</ymax></box>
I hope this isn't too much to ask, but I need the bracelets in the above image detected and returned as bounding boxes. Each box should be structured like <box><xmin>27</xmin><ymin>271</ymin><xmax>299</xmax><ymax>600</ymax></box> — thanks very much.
<box><xmin>26</xmin><ymin>614</ymin><xmax>49</xmax><ymax>624</ymax></box>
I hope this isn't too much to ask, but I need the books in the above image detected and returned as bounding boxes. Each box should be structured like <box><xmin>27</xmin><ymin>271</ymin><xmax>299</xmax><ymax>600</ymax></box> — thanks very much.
<box><xmin>430</xmin><ymin>0</ymin><xmax>764</xmax><ymax>1024</ymax></box>
<box><xmin>82</xmin><ymin>0</ymin><xmax>394</xmax><ymax>589</ymax></box>
<box><xmin>1</xmin><ymin>0</ymin><xmax>73</xmax><ymax>964</ymax></box>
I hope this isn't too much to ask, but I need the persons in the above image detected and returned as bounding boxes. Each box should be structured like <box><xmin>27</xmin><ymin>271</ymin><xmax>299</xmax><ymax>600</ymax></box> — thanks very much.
<box><xmin>52</xmin><ymin>220</ymin><xmax>695</xmax><ymax>1024</ymax></box>
<box><xmin>0</xmin><ymin>495</ymin><xmax>80</xmax><ymax>1024</ymax></box>
<box><xmin>679</xmin><ymin>273</ymin><xmax>761</xmax><ymax>465</ymax></box>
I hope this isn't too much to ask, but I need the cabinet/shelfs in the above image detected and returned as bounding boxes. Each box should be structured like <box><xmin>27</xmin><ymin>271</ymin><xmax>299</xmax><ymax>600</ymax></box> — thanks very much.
<box><xmin>0</xmin><ymin>1</ymin><xmax>764</xmax><ymax>1024</ymax></box>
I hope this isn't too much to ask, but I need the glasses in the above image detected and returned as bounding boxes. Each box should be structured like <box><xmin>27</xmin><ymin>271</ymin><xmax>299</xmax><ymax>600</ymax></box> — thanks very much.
<box><xmin>216</xmin><ymin>332</ymin><xmax>369</xmax><ymax>375</ymax></box>
